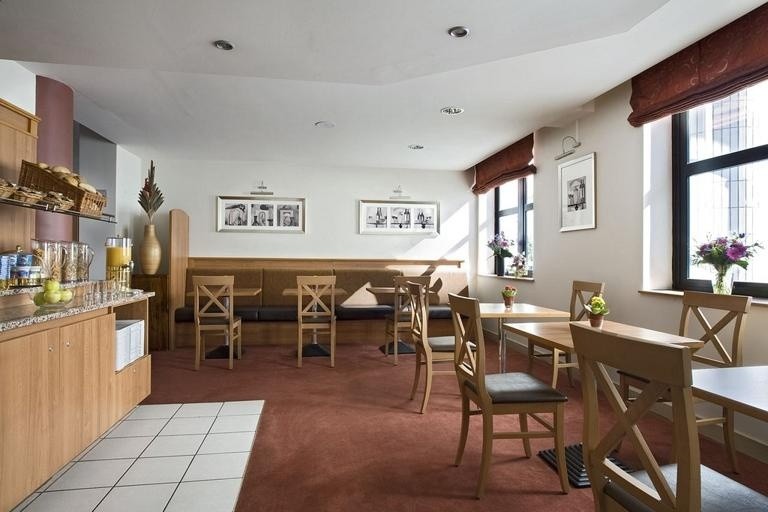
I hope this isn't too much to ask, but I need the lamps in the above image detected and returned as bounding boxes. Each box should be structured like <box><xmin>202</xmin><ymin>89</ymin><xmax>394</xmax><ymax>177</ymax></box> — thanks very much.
<box><xmin>553</xmin><ymin>133</ymin><xmax>582</xmax><ymax>160</ymax></box>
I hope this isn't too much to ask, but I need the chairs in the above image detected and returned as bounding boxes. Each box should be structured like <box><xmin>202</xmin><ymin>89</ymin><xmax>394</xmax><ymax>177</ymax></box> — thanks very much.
<box><xmin>294</xmin><ymin>274</ymin><xmax>337</xmax><ymax>370</ymax></box>
<box><xmin>442</xmin><ymin>290</ymin><xmax>571</xmax><ymax>496</ymax></box>
<box><xmin>526</xmin><ymin>281</ymin><xmax>606</xmax><ymax>387</ymax></box>
<box><xmin>618</xmin><ymin>290</ymin><xmax>755</xmax><ymax>477</ymax></box>
<box><xmin>556</xmin><ymin>320</ymin><xmax>766</xmax><ymax>512</ymax></box>
<box><xmin>384</xmin><ymin>275</ymin><xmax>430</xmax><ymax>369</ymax></box>
<box><xmin>192</xmin><ymin>275</ymin><xmax>241</xmax><ymax>370</ymax></box>
<box><xmin>401</xmin><ymin>279</ymin><xmax>475</xmax><ymax>414</ymax></box>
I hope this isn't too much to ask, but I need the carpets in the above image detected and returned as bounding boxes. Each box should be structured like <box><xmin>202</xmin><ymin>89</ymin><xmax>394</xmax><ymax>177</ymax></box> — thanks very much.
<box><xmin>135</xmin><ymin>338</ymin><xmax>765</xmax><ymax>510</ymax></box>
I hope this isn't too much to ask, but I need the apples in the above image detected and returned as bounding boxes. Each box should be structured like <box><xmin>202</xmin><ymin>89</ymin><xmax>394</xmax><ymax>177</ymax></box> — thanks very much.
<box><xmin>34</xmin><ymin>292</ymin><xmax>44</xmax><ymax>306</ymax></box>
<box><xmin>44</xmin><ymin>290</ymin><xmax>61</xmax><ymax>304</ymax></box>
<box><xmin>44</xmin><ymin>278</ymin><xmax>58</xmax><ymax>290</ymax></box>
<box><xmin>59</xmin><ymin>288</ymin><xmax>72</xmax><ymax>302</ymax></box>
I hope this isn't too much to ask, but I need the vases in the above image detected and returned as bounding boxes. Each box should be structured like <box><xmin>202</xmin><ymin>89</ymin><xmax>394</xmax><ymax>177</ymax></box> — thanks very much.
<box><xmin>504</xmin><ymin>297</ymin><xmax>515</xmax><ymax>310</ymax></box>
<box><xmin>494</xmin><ymin>255</ymin><xmax>504</xmax><ymax>276</ymax></box>
<box><xmin>711</xmin><ymin>274</ymin><xmax>732</xmax><ymax>294</ymax></box>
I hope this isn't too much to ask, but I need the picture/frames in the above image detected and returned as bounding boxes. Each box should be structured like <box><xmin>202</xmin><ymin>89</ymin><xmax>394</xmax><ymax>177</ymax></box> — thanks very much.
<box><xmin>215</xmin><ymin>194</ymin><xmax>309</xmax><ymax>236</ymax></box>
<box><xmin>356</xmin><ymin>198</ymin><xmax>442</xmax><ymax>238</ymax></box>
<box><xmin>555</xmin><ymin>152</ymin><xmax>597</xmax><ymax>234</ymax></box>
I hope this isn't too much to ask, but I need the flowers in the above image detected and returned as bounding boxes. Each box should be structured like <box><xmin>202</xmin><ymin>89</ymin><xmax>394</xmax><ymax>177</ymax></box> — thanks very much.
<box><xmin>508</xmin><ymin>252</ymin><xmax>530</xmax><ymax>277</ymax></box>
<box><xmin>690</xmin><ymin>232</ymin><xmax>766</xmax><ymax>290</ymax></box>
<box><xmin>499</xmin><ymin>285</ymin><xmax>518</xmax><ymax>297</ymax></box>
<box><xmin>486</xmin><ymin>227</ymin><xmax>515</xmax><ymax>258</ymax></box>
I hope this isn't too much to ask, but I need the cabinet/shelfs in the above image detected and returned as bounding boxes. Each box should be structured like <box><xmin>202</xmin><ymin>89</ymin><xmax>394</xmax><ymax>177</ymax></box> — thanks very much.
<box><xmin>131</xmin><ymin>270</ymin><xmax>171</xmax><ymax>353</ymax></box>
<box><xmin>113</xmin><ymin>294</ymin><xmax>154</xmax><ymax>421</ymax></box>
<box><xmin>1</xmin><ymin>310</ymin><xmax>115</xmax><ymax>512</ymax></box>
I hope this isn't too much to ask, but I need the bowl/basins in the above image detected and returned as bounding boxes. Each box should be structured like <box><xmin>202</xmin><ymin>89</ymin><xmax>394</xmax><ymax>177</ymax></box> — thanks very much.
<box><xmin>28</xmin><ymin>289</ymin><xmax>76</xmax><ymax>308</ymax></box>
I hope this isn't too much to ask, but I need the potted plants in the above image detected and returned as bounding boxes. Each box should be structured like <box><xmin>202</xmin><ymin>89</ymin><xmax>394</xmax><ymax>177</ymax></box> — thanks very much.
<box><xmin>137</xmin><ymin>155</ymin><xmax>166</xmax><ymax>277</ymax></box>
<box><xmin>583</xmin><ymin>296</ymin><xmax>610</xmax><ymax>331</ymax></box>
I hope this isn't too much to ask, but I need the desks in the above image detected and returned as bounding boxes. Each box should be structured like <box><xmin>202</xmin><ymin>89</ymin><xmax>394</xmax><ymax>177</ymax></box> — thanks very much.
<box><xmin>465</xmin><ymin>292</ymin><xmax>569</xmax><ymax>379</ymax></box>
<box><xmin>188</xmin><ymin>285</ymin><xmax>261</xmax><ymax>299</ymax></box>
<box><xmin>366</xmin><ymin>284</ymin><xmax>437</xmax><ymax>299</ymax></box>
<box><xmin>500</xmin><ymin>314</ymin><xmax>705</xmax><ymax>487</ymax></box>
<box><xmin>279</xmin><ymin>286</ymin><xmax>348</xmax><ymax>299</ymax></box>
<box><xmin>655</xmin><ymin>358</ymin><xmax>767</xmax><ymax>426</ymax></box>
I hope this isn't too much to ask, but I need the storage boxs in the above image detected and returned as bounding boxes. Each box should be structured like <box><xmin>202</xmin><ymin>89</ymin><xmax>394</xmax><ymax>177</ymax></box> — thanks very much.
<box><xmin>114</xmin><ymin>314</ymin><xmax>145</xmax><ymax>375</ymax></box>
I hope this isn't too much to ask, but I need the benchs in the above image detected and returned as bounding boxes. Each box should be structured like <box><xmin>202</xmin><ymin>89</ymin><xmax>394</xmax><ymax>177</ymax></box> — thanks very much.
<box><xmin>174</xmin><ymin>253</ymin><xmax>473</xmax><ymax>352</ymax></box>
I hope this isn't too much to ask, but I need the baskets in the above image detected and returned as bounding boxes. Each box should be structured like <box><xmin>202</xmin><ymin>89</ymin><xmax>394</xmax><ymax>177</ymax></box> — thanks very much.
<box><xmin>0</xmin><ymin>159</ymin><xmax>105</xmax><ymax>216</ymax></box>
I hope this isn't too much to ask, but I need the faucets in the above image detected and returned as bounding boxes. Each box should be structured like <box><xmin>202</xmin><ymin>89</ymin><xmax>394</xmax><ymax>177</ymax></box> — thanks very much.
<box><xmin>123</xmin><ymin>260</ymin><xmax>136</xmax><ymax>272</ymax></box>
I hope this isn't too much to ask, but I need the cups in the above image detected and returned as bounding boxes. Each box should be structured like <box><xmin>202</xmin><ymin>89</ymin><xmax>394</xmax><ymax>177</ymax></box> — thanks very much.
<box><xmin>81</xmin><ymin>279</ymin><xmax>115</xmax><ymax>306</ymax></box>
<box><xmin>30</xmin><ymin>237</ymin><xmax>97</xmax><ymax>282</ymax></box>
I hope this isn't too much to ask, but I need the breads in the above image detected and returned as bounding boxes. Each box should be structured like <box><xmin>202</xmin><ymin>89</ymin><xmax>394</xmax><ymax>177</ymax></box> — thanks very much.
<box><xmin>37</xmin><ymin>162</ymin><xmax>103</xmax><ymax>197</ymax></box>
